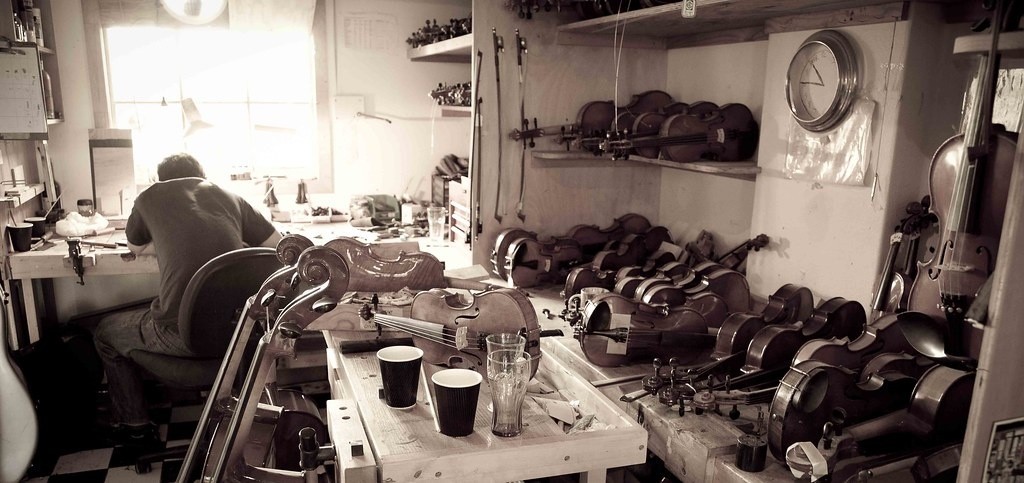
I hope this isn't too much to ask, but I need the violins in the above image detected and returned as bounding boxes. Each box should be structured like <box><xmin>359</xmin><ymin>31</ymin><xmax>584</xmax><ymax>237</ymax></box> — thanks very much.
<box><xmin>428</xmin><ymin>79</ymin><xmax>471</xmax><ymax>106</ymax></box>
<box><xmin>406</xmin><ymin>12</ymin><xmax>472</xmax><ymax>47</ymax></box>
<box><xmin>485</xmin><ymin>210</ymin><xmax>979</xmax><ymax>473</ymax></box>
<box><xmin>355</xmin><ymin>290</ymin><xmax>540</xmax><ymax>385</ymax></box>
<box><xmin>507</xmin><ymin>87</ymin><xmax>761</xmax><ymax>161</ymax></box>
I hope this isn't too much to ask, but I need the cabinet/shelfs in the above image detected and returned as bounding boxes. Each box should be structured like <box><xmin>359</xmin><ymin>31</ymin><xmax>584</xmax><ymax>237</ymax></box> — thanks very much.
<box><xmin>0</xmin><ymin>0</ymin><xmax>65</xmax><ymax>208</ymax></box>
<box><xmin>312</xmin><ymin>0</ymin><xmax>1024</xmax><ymax>483</ymax></box>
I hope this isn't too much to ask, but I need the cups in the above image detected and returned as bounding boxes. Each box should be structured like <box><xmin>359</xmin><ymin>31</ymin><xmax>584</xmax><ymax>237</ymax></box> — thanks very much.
<box><xmin>485</xmin><ymin>332</ymin><xmax>527</xmax><ymax>413</ymax></box>
<box><xmin>486</xmin><ymin>350</ymin><xmax>532</xmax><ymax>438</ymax></box>
<box><xmin>431</xmin><ymin>368</ymin><xmax>483</xmax><ymax>438</ymax></box>
<box><xmin>376</xmin><ymin>345</ymin><xmax>424</xmax><ymax>410</ymax></box>
<box><xmin>427</xmin><ymin>206</ymin><xmax>445</xmax><ymax>245</ymax></box>
<box><xmin>24</xmin><ymin>217</ymin><xmax>46</xmax><ymax>245</ymax></box>
<box><xmin>7</xmin><ymin>223</ymin><xmax>34</xmax><ymax>252</ymax></box>
<box><xmin>567</xmin><ymin>287</ymin><xmax>610</xmax><ymax>311</ymax></box>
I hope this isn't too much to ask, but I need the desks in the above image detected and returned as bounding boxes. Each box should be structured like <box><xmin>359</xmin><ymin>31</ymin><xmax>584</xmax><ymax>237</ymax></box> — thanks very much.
<box><xmin>0</xmin><ymin>215</ymin><xmax>471</xmax><ymax>344</ymax></box>
<box><xmin>317</xmin><ymin>329</ymin><xmax>647</xmax><ymax>483</ymax></box>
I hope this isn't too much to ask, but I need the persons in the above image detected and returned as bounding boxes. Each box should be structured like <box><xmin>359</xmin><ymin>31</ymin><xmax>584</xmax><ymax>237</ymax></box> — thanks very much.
<box><xmin>93</xmin><ymin>152</ymin><xmax>283</xmax><ymax>448</ymax></box>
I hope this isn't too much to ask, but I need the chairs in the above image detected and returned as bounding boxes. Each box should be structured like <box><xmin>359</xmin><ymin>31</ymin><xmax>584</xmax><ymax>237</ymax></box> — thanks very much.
<box><xmin>127</xmin><ymin>247</ymin><xmax>286</xmax><ymax>470</ymax></box>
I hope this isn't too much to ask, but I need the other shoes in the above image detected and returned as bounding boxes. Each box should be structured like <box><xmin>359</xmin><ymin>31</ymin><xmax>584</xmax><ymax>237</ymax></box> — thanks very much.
<box><xmin>124</xmin><ymin>422</ymin><xmax>153</xmax><ymax>444</ymax></box>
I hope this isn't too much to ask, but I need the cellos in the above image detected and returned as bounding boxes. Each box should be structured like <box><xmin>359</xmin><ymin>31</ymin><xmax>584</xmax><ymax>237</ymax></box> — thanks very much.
<box><xmin>203</xmin><ymin>246</ymin><xmax>352</xmax><ymax>483</ymax></box>
<box><xmin>168</xmin><ymin>234</ymin><xmax>312</xmax><ymax>483</ymax></box>
<box><xmin>903</xmin><ymin>0</ymin><xmax>1016</xmax><ymax>323</ymax></box>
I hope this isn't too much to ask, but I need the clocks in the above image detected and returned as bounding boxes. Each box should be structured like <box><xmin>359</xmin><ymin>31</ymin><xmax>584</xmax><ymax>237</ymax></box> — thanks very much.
<box><xmin>776</xmin><ymin>29</ymin><xmax>858</xmax><ymax>131</ymax></box>
